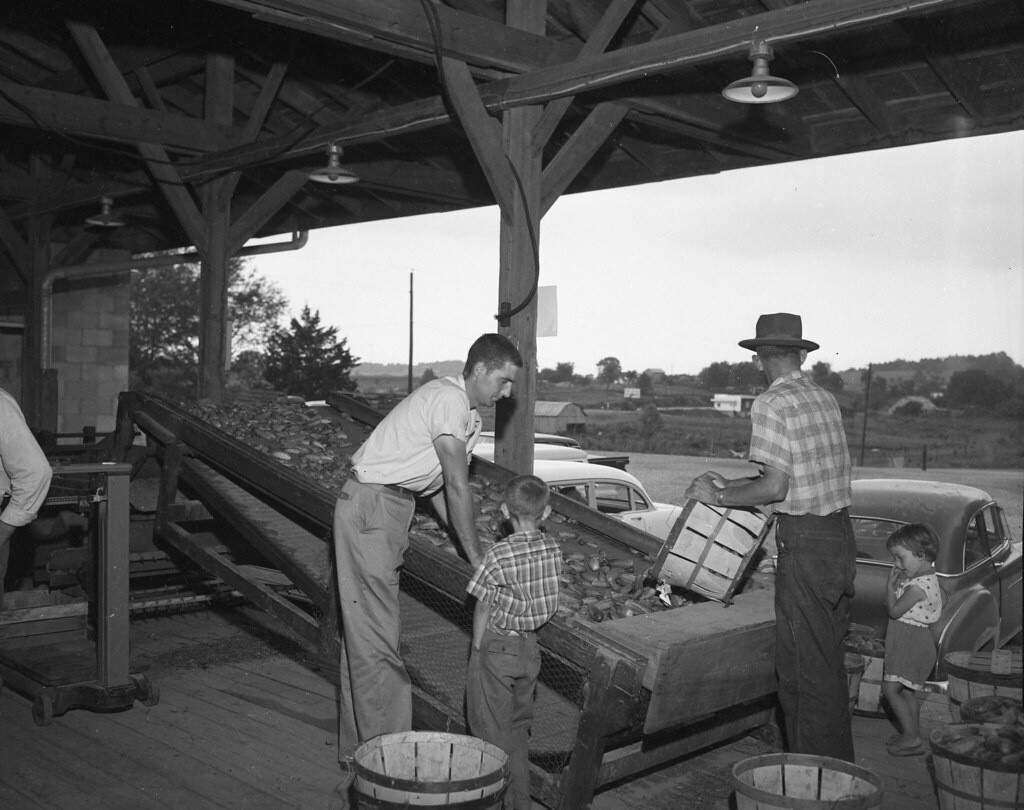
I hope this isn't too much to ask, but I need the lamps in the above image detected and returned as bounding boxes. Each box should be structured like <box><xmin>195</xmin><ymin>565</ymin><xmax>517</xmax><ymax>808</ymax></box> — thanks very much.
<box><xmin>86</xmin><ymin>199</ymin><xmax>126</xmax><ymax>227</ymax></box>
<box><xmin>309</xmin><ymin>148</ymin><xmax>358</xmax><ymax>185</ymax></box>
<box><xmin>721</xmin><ymin>44</ymin><xmax>799</xmax><ymax>105</ymax></box>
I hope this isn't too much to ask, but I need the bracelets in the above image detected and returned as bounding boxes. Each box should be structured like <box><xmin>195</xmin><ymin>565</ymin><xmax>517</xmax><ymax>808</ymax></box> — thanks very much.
<box><xmin>718</xmin><ymin>489</ymin><xmax>725</xmax><ymax>505</ymax></box>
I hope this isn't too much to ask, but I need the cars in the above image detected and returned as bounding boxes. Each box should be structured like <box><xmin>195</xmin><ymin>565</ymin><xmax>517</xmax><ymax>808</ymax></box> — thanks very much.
<box><xmin>462</xmin><ymin>432</ymin><xmax>685</xmax><ymax>543</ymax></box>
<box><xmin>836</xmin><ymin>478</ymin><xmax>1024</xmax><ymax>681</ymax></box>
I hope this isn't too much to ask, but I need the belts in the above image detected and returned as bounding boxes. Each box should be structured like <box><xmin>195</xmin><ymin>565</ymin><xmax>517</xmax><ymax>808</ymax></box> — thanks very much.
<box><xmin>384</xmin><ymin>482</ymin><xmax>417</xmax><ymax>496</ymax></box>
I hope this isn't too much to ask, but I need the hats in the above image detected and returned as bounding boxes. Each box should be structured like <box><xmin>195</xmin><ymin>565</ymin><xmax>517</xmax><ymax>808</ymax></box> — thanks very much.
<box><xmin>738</xmin><ymin>313</ymin><xmax>820</xmax><ymax>352</ymax></box>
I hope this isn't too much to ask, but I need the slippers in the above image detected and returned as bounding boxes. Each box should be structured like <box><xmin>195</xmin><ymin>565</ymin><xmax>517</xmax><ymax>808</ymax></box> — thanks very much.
<box><xmin>888</xmin><ymin>735</ymin><xmax>926</xmax><ymax>756</ymax></box>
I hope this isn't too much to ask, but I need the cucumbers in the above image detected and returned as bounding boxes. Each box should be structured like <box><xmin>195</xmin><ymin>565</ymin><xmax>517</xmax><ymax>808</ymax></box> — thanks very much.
<box><xmin>938</xmin><ymin>696</ymin><xmax>1024</xmax><ymax>770</ymax></box>
<box><xmin>842</xmin><ymin>635</ymin><xmax>886</xmax><ymax>653</ymax></box>
<box><xmin>168</xmin><ymin>395</ymin><xmax>695</xmax><ymax>623</ymax></box>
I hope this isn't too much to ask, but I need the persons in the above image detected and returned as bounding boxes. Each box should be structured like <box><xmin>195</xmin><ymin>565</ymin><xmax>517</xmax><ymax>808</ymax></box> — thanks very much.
<box><xmin>0</xmin><ymin>385</ymin><xmax>54</xmax><ymax>604</ymax></box>
<box><xmin>686</xmin><ymin>312</ymin><xmax>857</xmax><ymax>760</ymax></box>
<box><xmin>883</xmin><ymin>522</ymin><xmax>944</xmax><ymax>756</ymax></box>
<box><xmin>468</xmin><ymin>476</ymin><xmax>563</xmax><ymax>810</ymax></box>
<box><xmin>333</xmin><ymin>334</ymin><xmax>523</xmax><ymax>764</ymax></box>
